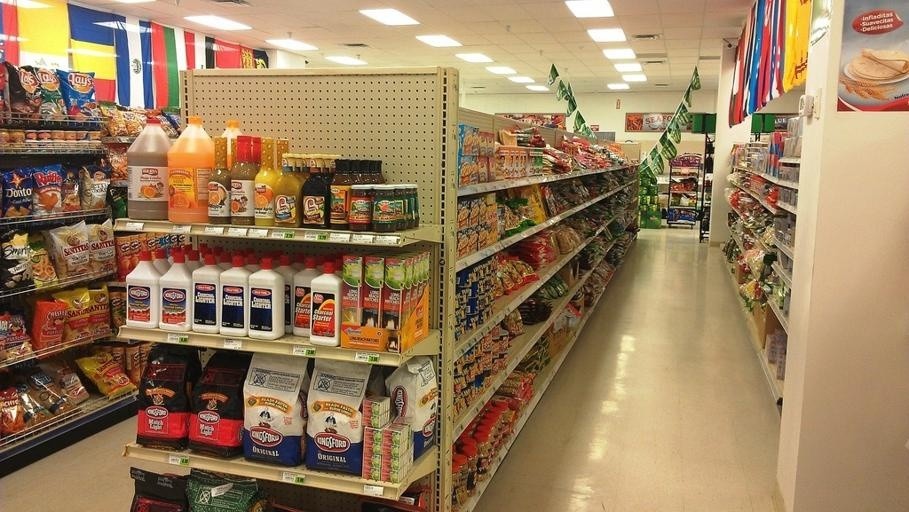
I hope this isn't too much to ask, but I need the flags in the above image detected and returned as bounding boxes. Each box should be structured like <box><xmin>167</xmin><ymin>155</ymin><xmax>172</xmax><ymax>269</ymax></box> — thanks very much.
<box><xmin>727</xmin><ymin>1</ymin><xmax>813</xmax><ymax>129</ymax></box>
<box><xmin>0</xmin><ymin>1</ymin><xmax>270</xmax><ymax>113</ymax></box>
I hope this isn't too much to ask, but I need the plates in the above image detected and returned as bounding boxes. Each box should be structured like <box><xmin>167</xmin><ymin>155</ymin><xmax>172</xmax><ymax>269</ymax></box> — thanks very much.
<box><xmin>844</xmin><ymin>62</ymin><xmax>908</xmax><ymax>83</ymax></box>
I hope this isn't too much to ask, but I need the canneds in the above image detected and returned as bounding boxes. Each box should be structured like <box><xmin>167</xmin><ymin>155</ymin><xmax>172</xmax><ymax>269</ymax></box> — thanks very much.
<box><xmin>0</xmin><ymin>129</ymin><xmax>102</xmax><ymax>152</ymax></box>
<box><xmin>348</xmin><ymin>183</ymin><xmax>419</xmax><ymax>231</ymax></box>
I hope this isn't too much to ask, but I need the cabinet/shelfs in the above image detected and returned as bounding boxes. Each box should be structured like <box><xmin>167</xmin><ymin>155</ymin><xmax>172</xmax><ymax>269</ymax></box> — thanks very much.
<box><xmin>106</xmin><ymin>211</ymin><xmax>444</xmax><ymax>510</ymax></box>
<box><xmin>719</xmin><ymin>142</ymin><xmax>804</xmax><ymax>420</ymax></box>
<box><xmin>100</xmin><ymin>132</ymin><xmax>175</xmax><ymax>412</ymax></box>
<box><xmin>665</xmin><ymin>151</ymin><xmax>700</xmax><ymax>232</ymax></box>
<box><xmin>0</xmin><ymin>109</ymin><xmax>180</xmax><ymax>478</ymax></box>
<box><xmin>452</xmin><ymin>159</ymin><xmax>642</xmax><ymax>510</ymax></box>
<box><xmin>698</xmin><ymin>128</ymin><xmax>715</xmax><ymax>246</ymax></box>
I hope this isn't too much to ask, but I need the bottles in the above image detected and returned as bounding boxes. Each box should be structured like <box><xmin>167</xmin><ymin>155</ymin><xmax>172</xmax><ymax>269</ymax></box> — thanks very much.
<box><xmin>124</xmin><ymin>243</ymin><xmax>342</xmax><ymax>349</ymax></box>
<box><xmin>123</xmin><ymin>111</ymin><xmax>419</xmax><ymax>233</ymax></box>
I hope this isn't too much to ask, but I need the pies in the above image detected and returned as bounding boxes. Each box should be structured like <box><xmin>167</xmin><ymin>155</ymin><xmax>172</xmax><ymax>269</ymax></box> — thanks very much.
<box><xmin>845</xmin><ymin>50</ymin><xmax>909</xmax><ymax>82</ymax></box>
